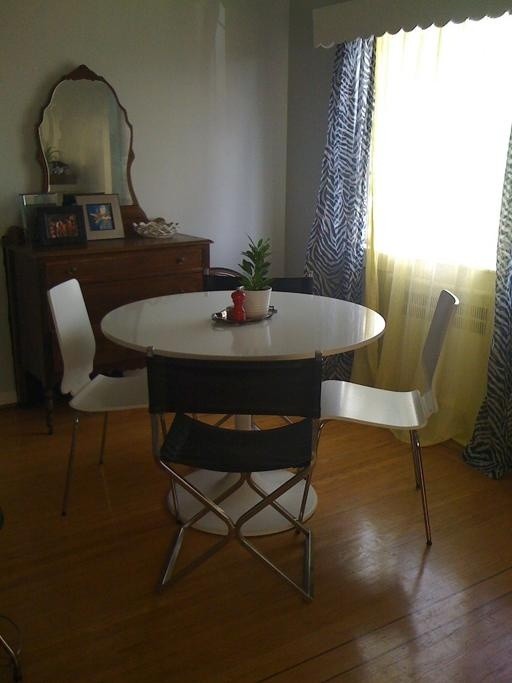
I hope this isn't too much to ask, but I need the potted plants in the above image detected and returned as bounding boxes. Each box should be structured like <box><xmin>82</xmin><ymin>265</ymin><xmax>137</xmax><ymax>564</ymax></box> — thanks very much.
<box><xmin>234</xmin><ymin>232</ymin><xmax>275</xmax><ymax>319</ymax></box>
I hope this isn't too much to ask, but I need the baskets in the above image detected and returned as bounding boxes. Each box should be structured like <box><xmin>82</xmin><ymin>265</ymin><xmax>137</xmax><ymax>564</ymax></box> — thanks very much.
<box><xmin>133</xmin><ymin>220</ymin><xmax>180</xmax><ymax>239</ymax></box>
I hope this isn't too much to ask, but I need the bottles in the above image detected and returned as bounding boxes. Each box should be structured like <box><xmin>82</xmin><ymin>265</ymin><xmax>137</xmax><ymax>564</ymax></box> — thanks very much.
<box><xmin>230</xmin><ymin>288</ymin><xmax>247</xmax><ymax>320</ymax></box>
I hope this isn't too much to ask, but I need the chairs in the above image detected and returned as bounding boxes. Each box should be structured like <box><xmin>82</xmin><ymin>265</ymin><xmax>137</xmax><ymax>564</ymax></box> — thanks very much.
<box><xmin>46</xmin><ymin>277</ymin><xmax>169</xmax><ymax>513</ymax></box>
<box><xmin>142</xmin><ymin>343</ymin><xmax>323</xmax><ymax>602</ymax></box>
<box><xmin>294</xmin><ymin>289</ymin><xmax>459</xmax><ymax>549</ymax></box>
<box><xmin>200</xmin><ymin>267</ymin><xmax>314</xmax><ymax>297</ymax></box>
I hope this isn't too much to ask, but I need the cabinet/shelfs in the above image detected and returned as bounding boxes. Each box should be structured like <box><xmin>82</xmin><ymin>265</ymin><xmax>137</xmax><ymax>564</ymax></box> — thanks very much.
<box><xmin>2</xmin><ymin>211</ymin><xmax>216</xmax><ymax>407</ymax></box>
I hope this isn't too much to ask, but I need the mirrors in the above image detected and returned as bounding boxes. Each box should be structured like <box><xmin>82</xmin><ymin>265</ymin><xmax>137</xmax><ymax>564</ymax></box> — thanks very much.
<box><xmin>33</xmin><ymin>61</ymin><xmax>139</xmax><ymax>206</ymax></box>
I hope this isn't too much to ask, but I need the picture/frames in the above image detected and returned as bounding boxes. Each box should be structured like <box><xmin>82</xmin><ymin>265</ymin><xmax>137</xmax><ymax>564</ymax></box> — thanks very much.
<box><xmin>19</xmin><ymin>192</ymin><xmax>125</xmax><ymax>250</ymax></box>
